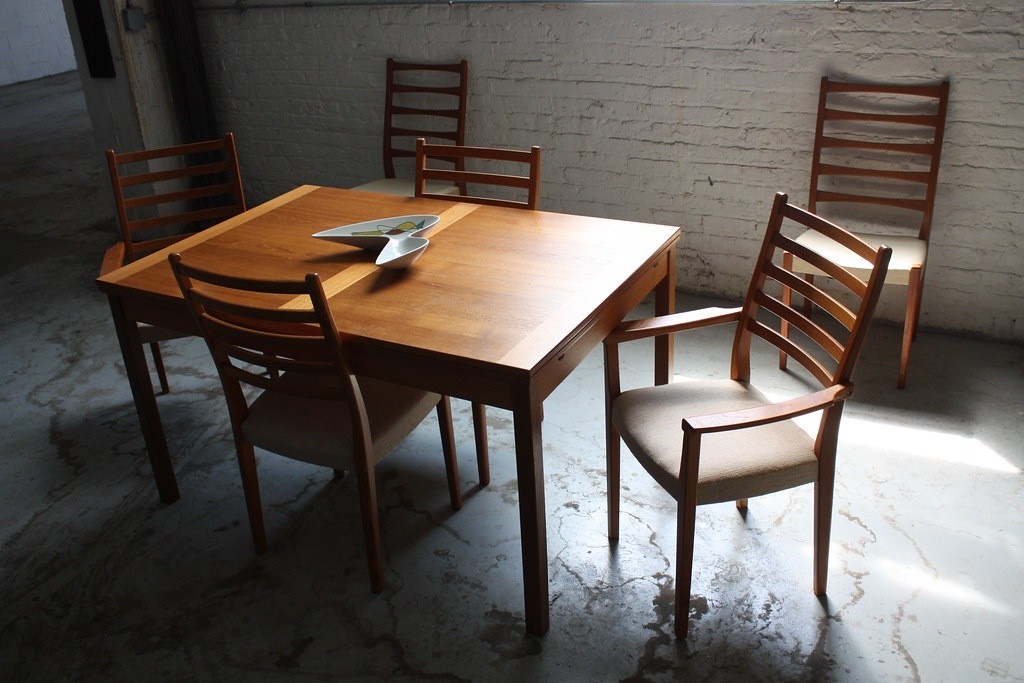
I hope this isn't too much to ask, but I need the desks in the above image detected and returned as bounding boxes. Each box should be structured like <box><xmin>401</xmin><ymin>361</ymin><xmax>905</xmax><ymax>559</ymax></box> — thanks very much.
<box><xmin>94</xmin><ymin>183</ymin><xmax>682</xmax><ymax>639</ymax></box>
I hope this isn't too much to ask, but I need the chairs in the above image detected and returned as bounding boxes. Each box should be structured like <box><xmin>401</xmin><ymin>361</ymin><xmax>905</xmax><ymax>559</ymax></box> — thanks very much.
<box><xmin>780</xmin><ymin>76</ymin><xmax>950</xmax><ymax>391</ymax></box>
<box><xmin>168</xmin><ymin>254</ymin><xmax>464</xmax><ymax>594</ymax></box>
<box><xmin>603</xmin><ymin>191</ymin><xmax>894</xmax><ymax>637</ymax></box>
<box><xmin>350</xmin><ymin>56</ymin><xmax>469</xmax><ymax>199</ymax></box>
<box><xmin>98</xmin><ymin>132</ymin><xmax>246</xmax><ymax>393</ymax></box>
<box><xmin>415</xmin><ymin>137</ymin><xmax>541</xmax><ymax>485</ymax></box>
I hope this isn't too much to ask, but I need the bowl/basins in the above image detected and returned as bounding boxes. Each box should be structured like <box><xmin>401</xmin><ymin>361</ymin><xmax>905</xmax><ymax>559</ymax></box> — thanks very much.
<box><xmin>311</xmin><ymin>214</ymin><xmax>439</xmax><ymax>271</ymax></box>
